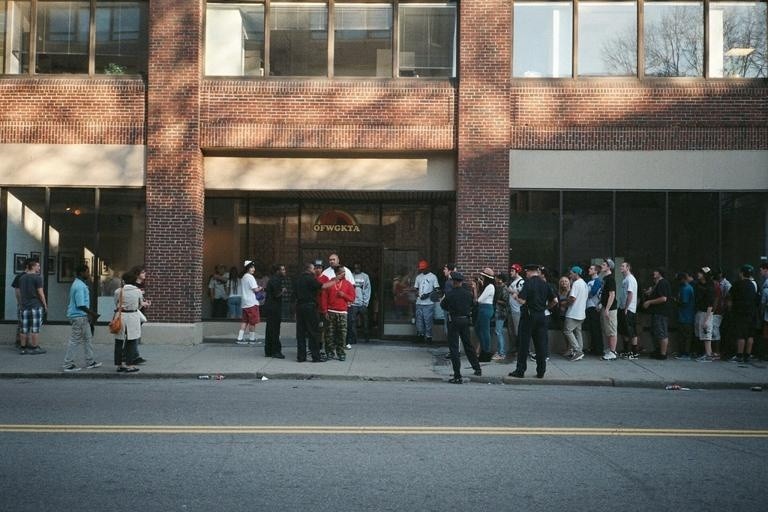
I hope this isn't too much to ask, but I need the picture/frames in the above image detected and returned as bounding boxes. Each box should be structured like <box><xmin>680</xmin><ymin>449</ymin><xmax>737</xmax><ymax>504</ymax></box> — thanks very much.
<box><xmin>12</xmin><ymin>248</ymin><xmax>113</xmax><ymax>284</ymax></box>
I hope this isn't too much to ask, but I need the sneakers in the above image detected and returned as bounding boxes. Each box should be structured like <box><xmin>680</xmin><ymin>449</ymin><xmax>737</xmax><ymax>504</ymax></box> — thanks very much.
<box><xmin>672</xmin><ymin>350</ymin><xmax>754</xmax><ymax>364</ymax></box>
<box><xmin>64</xmin><ymin>365</ymin><xmax>82</xmax><ymax>372</ymax></box>
<box><xmin>621</xmin><ymin>349</ymin><xmax>639</xmax><ymax>360</ymax></box>
<box><xmin>15</xmin><ymin>341</ymin><xmax>46</xmax><ymax>355</ymax></box>
<box><xmin>235</xmin><ymin>333</ymin><xmax>383</xmax><ymax>363</ymax></box>
<box><xmin>115</xmin><ymin>356</ymin><xmax>146</xmax><ymax>371</ymax></box>
<box><xmin>88</xmin><ymin>362</ymin><xmax>102</xmax><ymax>369</ymax></box>
<box><xmin>563</xmin><ymin>349</ymin><xmax>584</xmax><ymax>361</ymax></box>
<box><xmin>600</xmin><ymin>349</ymin><xmax>617</xmax><ymax>360</ymax></box>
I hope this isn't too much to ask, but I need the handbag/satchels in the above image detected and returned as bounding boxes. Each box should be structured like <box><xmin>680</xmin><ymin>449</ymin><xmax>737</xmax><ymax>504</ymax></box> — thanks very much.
<box><xmin>109</xmin><ymin>317</ymin><xmax>121</xmax><ymax>334</ymax></box>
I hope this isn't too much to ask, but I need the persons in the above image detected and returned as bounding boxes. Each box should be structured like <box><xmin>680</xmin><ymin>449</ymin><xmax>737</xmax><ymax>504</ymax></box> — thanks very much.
<box><xmin>209</xmin><ymin>255</ymin><xmax>371</xmax><ymax>362</ymax></box>
<box><xmin>10</xmin><ymin>258</ymin><xmax>48</xmax><ymax>354</ymax></box>
<box><xmin>63</xmin><ymin>264</ymin><xmax>103</xmax><ymax>371</ymax></box>
<box><xmin>110</xmin><ymin>265</ymin><xmax>152</xmax><ymax>372</ymax></box>
<box><xmin>414</xmin><ymin>256</ymin><xmax>768</xmax><ymax>382</ymax></box>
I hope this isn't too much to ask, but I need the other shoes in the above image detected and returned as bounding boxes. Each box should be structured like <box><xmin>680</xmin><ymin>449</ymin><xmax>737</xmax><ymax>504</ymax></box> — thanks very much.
<box><xmin>474</xmin><ymin>368</ymin><xmax>482</xmax><ymax>376</ymax></box>
<box><xmin>648</xmin><ymin>351</ymin><xmax>668</xmax><ymax>360</ymax></box>
<box><xmin>531</xmin><ymin>353</ymin><xmax>550</xmax><ymax>362</ymax></box>
<box><xmin>537</xmin><ymin>371</ymin><xmax>545</xmax><ymax>378</ymax></box>
<box><xmin>476</xmin><ymin>351</ymin><xmax>506</xmax><ymax>362</ymax></box>
<box><xmin>418</xmin><ymin>333</ymin><xmax>426</xmax><ymax>344</ymax></box>
<box><xmin>448</xmin><ymin>377</ymin><xmax>464</xmax><ymax>384</ymax></box>
<box><xmin>509</xmin><ymin>371</ymin><xmax>525</xmax><ymax>378</ymax></box>
<box><xmin>427</xmin><ymin>338</ymin><xmax>433</xmax><ymax>345</ymax></box>
<box><xmin>445</xmin><ymin>352</ymin><xmax>452</xmax><ymax>359</ymax></box>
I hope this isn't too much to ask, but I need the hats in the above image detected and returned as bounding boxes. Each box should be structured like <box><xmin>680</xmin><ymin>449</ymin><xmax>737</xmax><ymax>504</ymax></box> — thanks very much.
<box><xmin>244</xmin><ymin>260</ymin><xmax>255</xmax><ymax>268</ymax></box>
<box><xmin>511</xmin><ymin>264</ymin><xmax>524</xmax><ymax>273</ymax></box>
<box><xmin>605</xmin><ymin>258</ymin><xmax>615</xmax><ymax>270</ymax></box>
<box><xmin>653</xmin><ymin>263</ymin><xmax>754</xmax><ymax>280</ymax></box>
<box><xmin>571</xmin><ymin>265</ymin><xmax>583</xmax><ymax>275</ymax></box>
<box><xmin>449</xmin><ymin>273</ymin><xmax>465</xmax><ymax>282</ymax></box>
<box><xmin>445</xmin><ymin>263</ymin><xmax>455</xmax><ymax>271</ymax></box>
<box><xmin>481</xmin><ymin>268</ymin><xmax>494</xmax><ymax>280</ymax></box>
<box><xmin>525</xmin><ymin>263</ymin><xmax>540</xmax><ymax>270</ymax></box>
<box><xmin>418</xmin><ymin>261</ymin><xmax>429</xmax><ymax>270</ymax></box>
